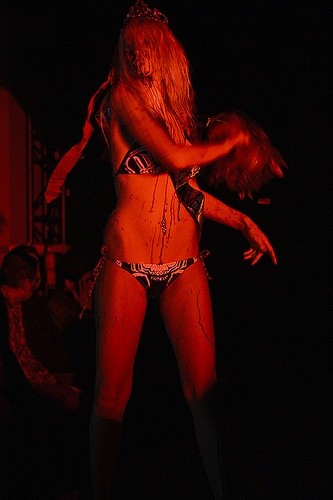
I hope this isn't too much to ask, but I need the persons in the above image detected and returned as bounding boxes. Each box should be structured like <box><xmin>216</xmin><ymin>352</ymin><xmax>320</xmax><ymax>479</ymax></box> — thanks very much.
<box><xmin>0</xmin><ymin>243</ymin><xmax>97</xmax><ymax>468</ymax></box>
<box><xmin>80</xmin><ymin>2</ymin><xmax>280</xmax><ymax>500</ymax></box>
